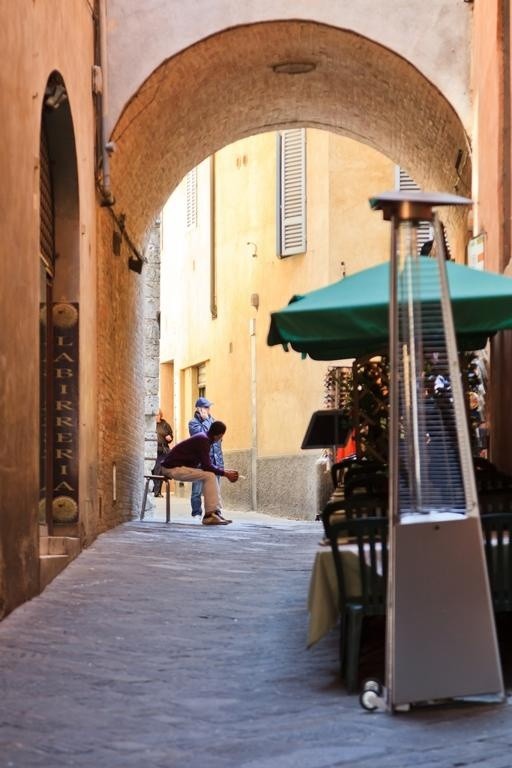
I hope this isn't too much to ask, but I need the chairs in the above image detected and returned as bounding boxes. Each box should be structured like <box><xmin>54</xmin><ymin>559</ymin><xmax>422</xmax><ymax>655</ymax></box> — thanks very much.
<box><xmin>471</xmin><ymin>453</ymin><xmax>511</xmax><ymax>686</ymax></box>
<box><xmin>308</xmin><ymin>454</ymin><xmax>389</xmax><ymax>693</ymax></box>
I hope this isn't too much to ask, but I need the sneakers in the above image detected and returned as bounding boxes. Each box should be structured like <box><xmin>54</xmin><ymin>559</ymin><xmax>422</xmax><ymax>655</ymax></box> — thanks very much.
<box><xmin>191</xmin><ymin>509</ymin><xmax>233</xmax><ymax>526</ymax></box>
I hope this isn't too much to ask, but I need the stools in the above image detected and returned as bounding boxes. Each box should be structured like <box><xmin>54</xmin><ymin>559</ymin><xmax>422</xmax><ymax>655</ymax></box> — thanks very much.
<box><xmin>140</xmin><ymin>475</ymin><xmax>173</xmax><ymax>523</ymax></box>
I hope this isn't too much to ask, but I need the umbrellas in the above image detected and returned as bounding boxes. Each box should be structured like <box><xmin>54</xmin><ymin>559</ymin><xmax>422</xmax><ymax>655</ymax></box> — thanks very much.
<box><xmin>267</xmin><ymin>253</ymin><xmax>512</xmax><ymax>361</ymax></box>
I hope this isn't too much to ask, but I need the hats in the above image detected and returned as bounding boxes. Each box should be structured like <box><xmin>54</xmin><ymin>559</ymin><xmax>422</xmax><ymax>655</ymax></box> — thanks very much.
<box><xmin>194</xmin><ymin>398</ymin><xmax>214</xmax><ymax>408</ymax></box>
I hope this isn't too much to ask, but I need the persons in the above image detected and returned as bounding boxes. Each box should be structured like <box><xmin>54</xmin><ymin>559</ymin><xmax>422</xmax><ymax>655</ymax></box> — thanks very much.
<box><xmin>159</xmin><ymin>419</ymin><xmax>238</xmax><ymax>525</ymax></box>
<box><xmin>189</xmin><ymin>398</ymin><xmax>225</xmax><ymax>519</ymax></box>
<box><xmin>153</xmin><ymin>408</ymin><xmax>174</xmax><ymax>496</ymax></box>
